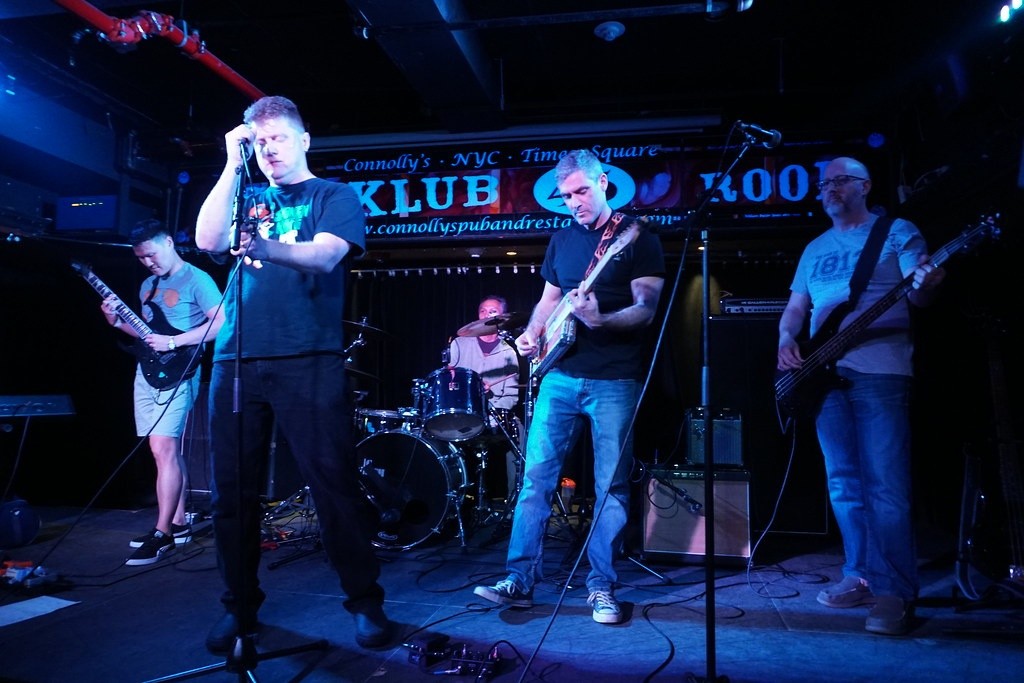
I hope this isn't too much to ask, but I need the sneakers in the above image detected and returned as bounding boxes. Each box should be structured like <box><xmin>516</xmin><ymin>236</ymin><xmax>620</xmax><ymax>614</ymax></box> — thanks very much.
<box><xmin>129</xmin><ymin>522</ymin><xmax>193</xmax><ymax>547</ymax></box>
<box><xmin>586</xmin><ymin>590</ymin><xmax>623</xmax><ymax>623</ymax></box>
<box><xmin>473</xmin><ymin>579</ymin><xmax>534</xmax><ymax>608</ymax></box>
<box><xmin>125</xmin><ymin>528</ymin><xmax>177</xmax><ymax>566</ymax></box>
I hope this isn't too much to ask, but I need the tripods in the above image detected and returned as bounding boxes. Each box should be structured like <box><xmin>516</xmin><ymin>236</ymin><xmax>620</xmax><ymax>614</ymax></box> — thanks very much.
<box><xmin>141</xmin><ymin>154</ymin><xmax>330</xmax><ymax>683</ymax></box>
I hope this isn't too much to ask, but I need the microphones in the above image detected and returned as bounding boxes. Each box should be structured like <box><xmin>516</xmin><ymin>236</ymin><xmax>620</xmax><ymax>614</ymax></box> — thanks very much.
<box><xmin>736</xmin><ymin>120</ymin><xmax>781</xmax><ymax>148</ymax></box>
<box><xmin>691</xmin><ymin>502</ymin><xmax>702</xmax><ymax>512</ymax></box>
<box><xmin>240</xmin><ymin>125</ymin><xmax>251</xmax><ymax>146</ymax></box>
<box><xmin>444</xmin><ymin>337</ymin><xmax>454</xmax><ymax>365</ymax></box>
<box><xmin>382</xmin><ymin>509</ymin><xmax>401</xmax><ymax>522</ymax></box>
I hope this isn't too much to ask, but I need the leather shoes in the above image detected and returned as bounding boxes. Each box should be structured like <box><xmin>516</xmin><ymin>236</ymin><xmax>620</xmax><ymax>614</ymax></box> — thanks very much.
<box><xmin>354</xmin><ymin>605</ymin><xmax>393</xmax><ymax>648</ymax></box>
<box><xmin>205</xmin><ymin>609</ymin><xmax>257</xmax><ymax>652</ymax></box>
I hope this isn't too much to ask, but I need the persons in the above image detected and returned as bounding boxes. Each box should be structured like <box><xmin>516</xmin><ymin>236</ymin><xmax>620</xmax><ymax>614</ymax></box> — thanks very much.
<box><xmin>194</xmin><ymin>96</ymin><xmax>389</xmax><ymax>653</ymax></box>
<box><xmin>445</xmin><ymin>299</ymin><xmax>520</xmax><ymax>521</ymax></box>
<box><xmin>472</xmin><ymin>147</ymin><xmax>664</xmax><ymax>624</ymax></box>
<box><xmin>100</xmin><ymin>225</ymin><xmax>224</xmax><ymax>565</ymax></box>
<box><xmin>778</xmin><ymin>158</ymin><xmax>946</xmax><ymax>636</ymax></box>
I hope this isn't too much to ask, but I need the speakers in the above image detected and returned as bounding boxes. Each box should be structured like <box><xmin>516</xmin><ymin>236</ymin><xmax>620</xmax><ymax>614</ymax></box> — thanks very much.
<box><xmin>0</xmin><ymin>499</ymin><xmax>42</xmax><ymax>551</ymax></box>
<box><xmin>639</xmin><ymin>463</ymin><xmax>755</xmax><ymax>570</ymax></box>
<box><xmin>700</xmin><ymin>312</ymin><xmax>833</xmax><ymax>540</ymax></box>
<box><xmin>685</xmin><ymin>409</ymin><xmax>745</xmax><ymax>468</ymax></box>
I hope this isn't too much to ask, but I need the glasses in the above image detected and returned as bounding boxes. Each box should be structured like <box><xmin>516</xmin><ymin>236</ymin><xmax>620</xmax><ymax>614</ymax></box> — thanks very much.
<box><xmin>815</xmin><ymin>175</ymin><xmax>866</xmax><ymax>191</ymax></box>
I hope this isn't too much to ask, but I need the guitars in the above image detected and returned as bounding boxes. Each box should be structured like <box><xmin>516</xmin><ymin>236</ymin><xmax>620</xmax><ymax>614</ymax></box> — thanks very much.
<box><xmin>69</xmin><ymin>256</ymin><xmax>204</xmax><ymax>391</ymax></box>
<box><xmin>774</xmin><ymin>212</ymin><xmax>1003</xmax><ymax>421</ymax></box>
<box><xmin>529</xmin><ymin>222</ymin><xmax>640</xmax><ymax>377</ymax></box>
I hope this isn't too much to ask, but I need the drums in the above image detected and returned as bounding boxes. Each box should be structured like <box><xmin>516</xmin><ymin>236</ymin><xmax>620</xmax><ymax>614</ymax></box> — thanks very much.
<box><xmin>353</xmin><ymin>406</ymin><xmax>412</xmax><ymax>438</ymax></box>
<box><xmin>355</xmin><ymin>425</ymin><xmax>467</xmax><ymax>552</ymax></box>
<box><xmin>418</xmin><ymin>367</ymin><xmax>489</xmax><ymax>442</ymax></box>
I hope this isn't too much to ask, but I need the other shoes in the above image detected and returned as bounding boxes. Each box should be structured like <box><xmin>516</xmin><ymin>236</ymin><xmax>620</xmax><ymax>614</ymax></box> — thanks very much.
<box><xmin>816</xmin><ymin>574</ymin><xmax>877</xmax><ymax>607</ymax></box>
<box><xmin>866</xmin><ymin>596</ymin><xmax>916</xmax><ymax>634</ymax></box>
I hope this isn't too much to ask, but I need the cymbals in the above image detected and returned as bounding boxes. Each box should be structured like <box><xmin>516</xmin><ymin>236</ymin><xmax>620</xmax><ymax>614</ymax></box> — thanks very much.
<box><xmin>456</xmin><ymin>311</ymin><xmax>533</xmax><ymax>337</ymax></box>
<box><xmin>341</xmin><ymin>319</ymin><xmax>399</xmax><ymax>343</ymax></box>
<box><xmin>343</xmin><ymin>367</ymin><xmax>384</xmax><ymax>384</ymax></box>
